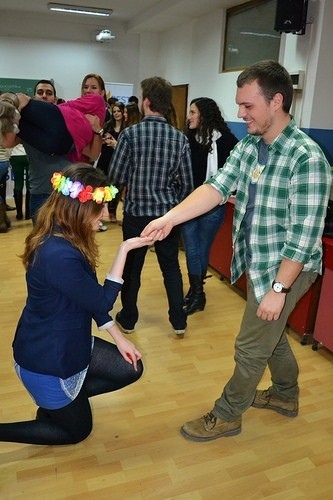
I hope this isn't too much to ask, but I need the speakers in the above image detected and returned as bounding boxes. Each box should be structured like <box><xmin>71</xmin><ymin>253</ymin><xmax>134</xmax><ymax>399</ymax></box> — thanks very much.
<box><xmin>274</xmin><ymin>0</ymin><xmax>305</xmax><ymax>32</ymax></box>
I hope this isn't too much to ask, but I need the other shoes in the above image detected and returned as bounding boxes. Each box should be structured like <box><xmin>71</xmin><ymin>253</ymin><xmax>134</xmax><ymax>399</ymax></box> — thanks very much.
<box><xmin>173</xmin><ymin>326</ymin><xmax>186</xmax><ymax>334</ymax></box>
<box><xmin>7</xmin><ymin>204</ymin><xmax>16</xmax><ymax>210</ymax></box>
<box><xmin>117</xmin><ymin>311</ymin><xmax>134</xmax><ymax>333</ymax></box>
<box><xmin>109</xmin><ymin>210</ymin><xmax>117</xmax><ymax>222</ymax></box>
<box><xmin>96</xmin><ymin>222</ymin><xmax>107</xmax><ymax>231</ymax></box>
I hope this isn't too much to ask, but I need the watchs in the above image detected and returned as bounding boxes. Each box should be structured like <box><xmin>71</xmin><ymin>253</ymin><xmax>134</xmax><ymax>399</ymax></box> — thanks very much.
<box><xmin>93</xmin><ymin>128</ymin><xmax>104</xmax><ymax>135</ymax></box>
<box><xmin>272</xmin><ymin>279</ymin><xmax>291</xmax><ymax>294</ymax></box>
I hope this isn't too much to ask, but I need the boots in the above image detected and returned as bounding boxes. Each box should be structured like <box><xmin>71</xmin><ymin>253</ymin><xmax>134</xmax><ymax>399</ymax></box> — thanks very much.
<box><xmin>0</xmin><ymin>201</ymin><xmax>11</xmax><ymax>232</ymax></box>
<box><xmin>183</xmin><ymin>276</ymin><xmax>205</xmax><ymax>305</ymax></box>
<box><xmin>184</xmin><ymin>274</ymin><xmax>206</xmax><ymax>314</ymax></box>
<box><xmin>13</xmin><ymin>188</ymin><xmax>23</xmax><ymax>219</ymax></box>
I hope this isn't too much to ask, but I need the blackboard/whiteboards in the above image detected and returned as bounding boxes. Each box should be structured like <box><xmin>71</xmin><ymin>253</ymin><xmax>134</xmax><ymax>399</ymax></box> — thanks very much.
<box><xmin>0</xmin><ymin>78</ymin><xmax>55</xmax><ymax>98</ymax></box>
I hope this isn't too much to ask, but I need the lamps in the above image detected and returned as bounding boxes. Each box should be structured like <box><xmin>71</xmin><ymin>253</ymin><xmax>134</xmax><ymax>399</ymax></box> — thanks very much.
<box><xmin>48</xmin><ymin>2</ymin><xmax>113</xmax><ymax>16</ymax></box>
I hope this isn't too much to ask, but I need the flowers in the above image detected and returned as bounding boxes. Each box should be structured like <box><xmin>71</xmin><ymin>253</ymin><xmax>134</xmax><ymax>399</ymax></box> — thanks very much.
<box><xmin>47</xmin><ymin>173</ymin><xmax>119</xmax><ymax>204</ymax></box>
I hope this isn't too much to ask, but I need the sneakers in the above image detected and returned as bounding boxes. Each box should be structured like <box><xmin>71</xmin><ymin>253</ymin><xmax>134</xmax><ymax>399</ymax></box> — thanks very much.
<box><xmin>252</xmin><ymin>389</ymin><xmax>299</xmax><ymax>416</ymax></box>
<box><xmin>182</xmin><ymin>410</ymin><xmax>243</xmax><ymax>441</ymax></box>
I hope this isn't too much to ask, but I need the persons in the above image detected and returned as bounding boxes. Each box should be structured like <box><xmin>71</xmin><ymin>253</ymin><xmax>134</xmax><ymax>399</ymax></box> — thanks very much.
<box><xmin>143</xmin><ymin>60</ymin><xmax>332</xmax><ymax>442</ymax></box>
<box><xmin>0</xmin><ymin>162</ymin><xmax>163</xmax><ymax>445</ymax></box>
<box><xmin>0</xmin><ymin>73</ymin><xmax>239</xmax><ymax>335</ymax></box>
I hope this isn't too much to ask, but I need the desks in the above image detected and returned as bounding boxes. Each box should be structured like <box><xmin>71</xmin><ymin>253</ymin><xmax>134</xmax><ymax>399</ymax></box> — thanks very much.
<box><xmin>207</xmin><ymin>194</ymin><xmax>333</xmax><ymax>361</ymax></box>
